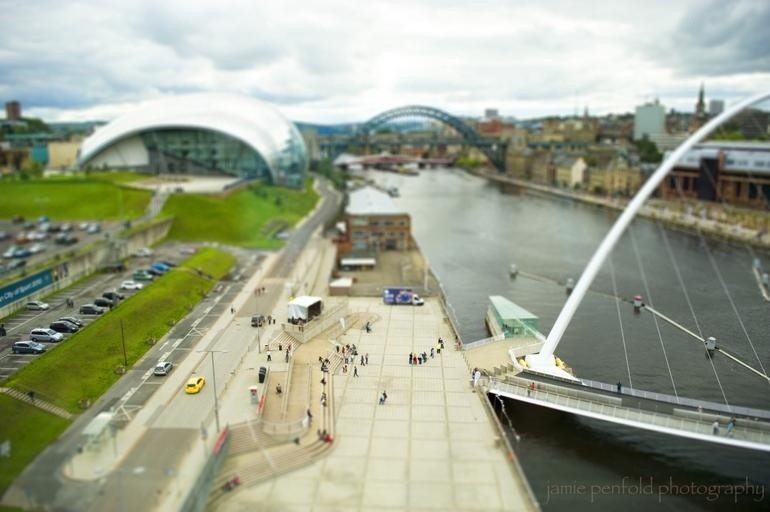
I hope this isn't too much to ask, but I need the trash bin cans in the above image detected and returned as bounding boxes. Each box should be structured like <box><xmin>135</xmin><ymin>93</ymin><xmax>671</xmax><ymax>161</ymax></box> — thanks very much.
<box><xmin>259</xmin><ymin>367</ymin><xmax>266</xmax><ymax>382</ymax></box>
<box><xmin>265</xmin><ymin>345</ymin><xmax>269</xmax><ymax>350</ymax></box>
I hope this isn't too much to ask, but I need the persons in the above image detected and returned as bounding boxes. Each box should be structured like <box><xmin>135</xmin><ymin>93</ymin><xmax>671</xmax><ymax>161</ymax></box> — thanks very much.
<box><xmin>408</xmin><ymin>338</ymin><xmax>445</xmax><ymax>366</ymax></box>
<box><xmin>472</xmin><ymin>367</ymin><xmax>481</xmax><ymax>393</ymax></box>
<box><xmin>379</xmin><ymin>392</ymin><xmax>385</xmax><ymax>405</ymax></box>
<box><xmin>724</xmin><ymin>416</ymin><xmax>737</xmax><ymax>439</ymax></box>
<box><xmin>527</xmin><ymin>383</ymin><xmax>532</xmax><ymax>397</ymax></box>
<box><xmin>265</xmin><ymin>311</ymin><xmax>371</xmax><ymax>446</ymax></box>
<box><xmin>616</xmin><ymin>379</ymin><xmax>624</xmax><ymax>395</ymax></box>
<box><xmin>382</xmin><ymin>390</ymin><xmax>388</xmax><ymax>400</ymax></box>
<box><xmin>712</xmin><ymin>416</ymin><xmax>720</xmax><ymax>437</ymax></box>
<box><xmin>531</xmin><ymin>382</ymin><xmax>537</xmax><ymax>395</ymax></box>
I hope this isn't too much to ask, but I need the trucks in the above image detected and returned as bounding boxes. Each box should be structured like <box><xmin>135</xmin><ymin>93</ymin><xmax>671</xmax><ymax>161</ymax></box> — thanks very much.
<box><xmin>384</xmin><ymin>287</ymin><xmax>424</xmax><ymax>306</ymax></box>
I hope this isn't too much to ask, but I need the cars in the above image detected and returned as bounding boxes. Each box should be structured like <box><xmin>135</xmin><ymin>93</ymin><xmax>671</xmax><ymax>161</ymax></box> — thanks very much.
<box><xmin>251</xmin><ymin>315</ymin><xmax>262</xmax><ymax>327</ymax></box>
<box><xmin>12</xmin><ymin>261</ymin><xmax>170</xmax><ymax>355</ymax></box>
<box><xmin>184</xmin><ymin>374</ymin><xmax>204</xmax><ymax>394</ymax></box>
<box><xmin>0</xmin><ymin>215</ymin><xmax>102</xmax><ymax>274</ymax></box>
<box><xmin>154</xmin><ymin>361</ymin><xmax>171</xmax><ymax>377</ymax></box>
